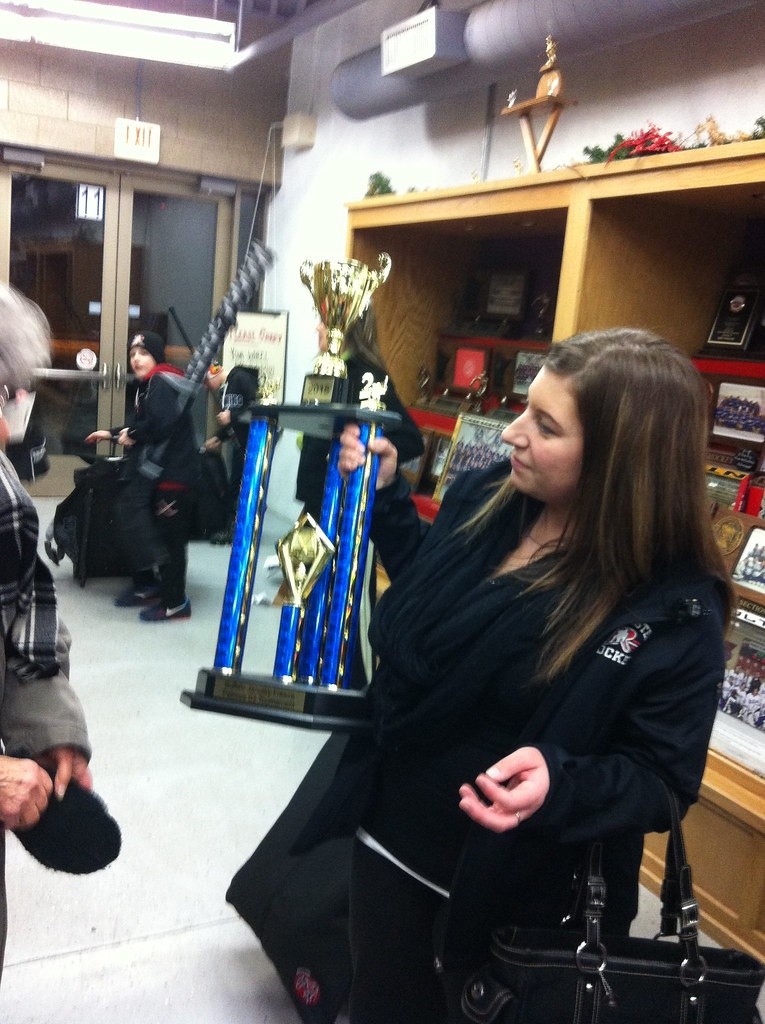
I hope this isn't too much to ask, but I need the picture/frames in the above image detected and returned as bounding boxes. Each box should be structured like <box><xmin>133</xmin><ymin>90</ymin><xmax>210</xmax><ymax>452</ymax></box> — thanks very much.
<box><xmin>430</xmin><ymin>411</ymin><xmax>513</xmax><ymax>502</ymax></box>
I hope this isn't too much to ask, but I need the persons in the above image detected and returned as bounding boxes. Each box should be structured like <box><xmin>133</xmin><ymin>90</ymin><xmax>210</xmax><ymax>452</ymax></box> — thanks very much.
<box><xmin>512</xmin><ymin>363</ymin><xmax>541</xmax><ymax>385</ymax></box>
<box><xmin>222</xmin><ymin>324</ymin><xmax>734</xmax><ymax>1024</ymax></box>
<box><xmin>719</xmin><ymin>665</ymin><xmax>765</xmax><ymax>732</ymax></box>
<box><xmin>82</xmin><ymin>330</ymin><xmax>199</xmax><ymax>622</ymax></box>
<box><xmin>738</xmin><ymin>543</ymin><xmax>765</xmax><ymax>586</ymax></box>
<box><xmin>444</xmin><ymin>439</ymin><xmax>512</xmax><ymax>485</ymax></box>
<box><xmin>202</xmin><ymin>359</ymin><xmax>261</xmax><ymax>545</ymax></box>
<box><xmin>715</xmin><ymin>395</ymin><xmax>765</xmax><ymax>433</ymax></box>
<box><xmin>0</xmin><ymin>274</ymin><xmax>94</xmax><ymax>968</ymax></box>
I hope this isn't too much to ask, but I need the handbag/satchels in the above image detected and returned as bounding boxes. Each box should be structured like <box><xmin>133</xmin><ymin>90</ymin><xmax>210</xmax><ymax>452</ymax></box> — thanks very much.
<box><xmin>452</xmin><ymin>814</ymin><xmax>765</xmax><ymax>1024</ymax></box>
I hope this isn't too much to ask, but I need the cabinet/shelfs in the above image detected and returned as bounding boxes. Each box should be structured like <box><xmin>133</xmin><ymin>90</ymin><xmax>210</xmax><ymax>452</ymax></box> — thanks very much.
<box><xmin>344</xmin><ymin>133</ymin><xmax>765</xmax><ymax>968</ymax></box>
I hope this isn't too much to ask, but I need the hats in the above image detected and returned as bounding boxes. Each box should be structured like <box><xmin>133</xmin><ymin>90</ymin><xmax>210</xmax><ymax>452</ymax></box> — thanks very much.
<box><xmin>131</xmin><ymin>330</ymin><xmax>164</xmax><ymax>363</ymax></box>
<box><xmin>204</xmin><ymin>359</ymin><xmax>223</xmax><ymax>380</ymax></box>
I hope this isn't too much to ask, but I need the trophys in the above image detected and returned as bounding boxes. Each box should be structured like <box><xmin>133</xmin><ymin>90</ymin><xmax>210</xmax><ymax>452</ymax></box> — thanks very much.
<box><xmin>446</xmin><ymin>261</ymin><xmax>549</xmax><ymax>340</ymax></box>
<box><xmin>183</xmin><ymin>249</ymin><xmax>402</xmax><ymax>731</ymax></box>
<box><xmin>413</xmin><ymin>344</ymin><xmax>491</xmax><ymax>420</ymax></box>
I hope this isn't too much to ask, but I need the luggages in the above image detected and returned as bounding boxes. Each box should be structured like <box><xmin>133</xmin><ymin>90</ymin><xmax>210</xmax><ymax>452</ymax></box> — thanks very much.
<box><xmin>72</xmin><ymin>435</ymin><xmax>152</xmax><ymax>587</ymax></box>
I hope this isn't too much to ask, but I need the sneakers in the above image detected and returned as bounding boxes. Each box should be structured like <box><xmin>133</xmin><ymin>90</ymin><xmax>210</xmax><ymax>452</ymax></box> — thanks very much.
<box><xmin>116</xmin><ymin>584</ymin><xmax>159</xmax><ymax>607</ymax></box>
<box><xmin>140</xmin><ymin>596</ymin><xmax>192</xmax><ymax>621</ymax></box>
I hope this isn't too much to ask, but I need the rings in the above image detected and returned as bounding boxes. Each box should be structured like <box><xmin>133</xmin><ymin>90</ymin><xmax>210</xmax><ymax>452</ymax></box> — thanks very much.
<box><xmin>515</xmin><ymin>811</ymin><xmax>522</xmax><ymax>825</ymax></box>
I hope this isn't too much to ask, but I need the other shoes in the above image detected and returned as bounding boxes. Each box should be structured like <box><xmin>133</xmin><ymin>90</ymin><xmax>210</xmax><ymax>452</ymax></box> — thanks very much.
<box><xmin>210</xmin><ymin>529</ymin><xmax>230</xmax><ymax>544</ymax></box>
